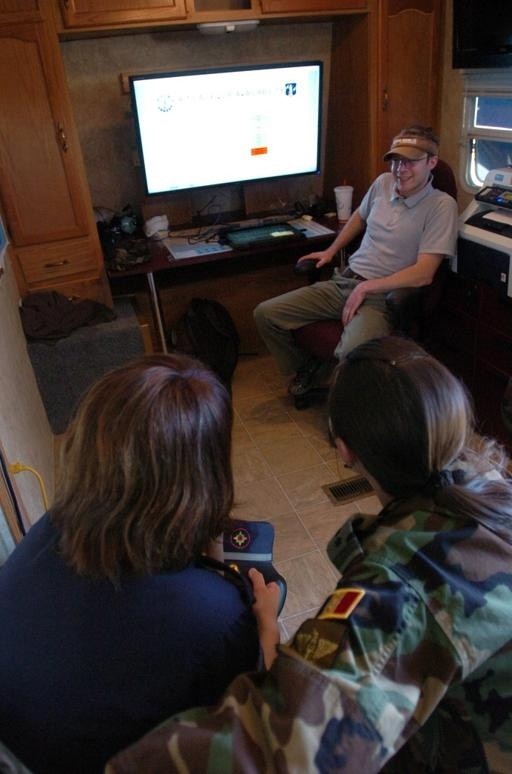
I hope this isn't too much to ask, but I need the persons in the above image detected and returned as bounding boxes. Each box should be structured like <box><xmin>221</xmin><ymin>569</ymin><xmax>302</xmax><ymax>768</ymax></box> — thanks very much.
<box><xmin>252</xmin><ymin>126</ymin><xmax>461</xmax><ymax>447</ymax></box>
<box><xmin>2</xmin><ymin>354</ymin><xmax>281</xmax><ymax>774</ymax></box>
<box><xmin>109</xmin><ymin>335</ymin><xmax>512</xmax><ymax>773</ymax></box>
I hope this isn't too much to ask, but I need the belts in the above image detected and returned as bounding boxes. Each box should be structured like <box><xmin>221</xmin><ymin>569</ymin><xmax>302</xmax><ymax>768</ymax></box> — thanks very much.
<box><xmin>345</xmin><ymin>268</ymin><xmax>368</xmax><ymax>282</ymax></box>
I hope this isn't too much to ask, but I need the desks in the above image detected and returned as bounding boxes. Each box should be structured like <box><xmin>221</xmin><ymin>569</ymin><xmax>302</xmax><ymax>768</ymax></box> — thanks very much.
<box><xmin>106</xmin><ymin>207</ymin><xmax>346</xmax><ymax>352</ymax></box>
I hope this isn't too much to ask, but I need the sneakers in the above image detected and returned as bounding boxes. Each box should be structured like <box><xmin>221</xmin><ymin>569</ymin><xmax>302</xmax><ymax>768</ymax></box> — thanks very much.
<box><xmin>288</xmin><ymin>371</ymin><xmax>316</xmax><ymax>394</ymax></box>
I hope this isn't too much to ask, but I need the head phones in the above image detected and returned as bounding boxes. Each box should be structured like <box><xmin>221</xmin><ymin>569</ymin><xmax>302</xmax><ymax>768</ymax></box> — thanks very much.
<box><xmin>111</xmin><ymin>209</ymin><xmax>141</xmax><ymax>236</ymax></box>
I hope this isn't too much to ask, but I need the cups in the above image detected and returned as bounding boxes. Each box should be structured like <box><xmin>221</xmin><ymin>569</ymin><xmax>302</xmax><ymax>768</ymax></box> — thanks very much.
<box><xmin>334</xmin><ymin>186</ymin><xmax>354</xmax><ymax>221</ymax></box>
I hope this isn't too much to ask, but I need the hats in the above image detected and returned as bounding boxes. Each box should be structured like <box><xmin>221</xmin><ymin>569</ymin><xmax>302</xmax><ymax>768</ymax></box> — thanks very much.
<box><xmin>383</xmin><ymin>126</ymin><xmax>439</xmax><ymax>161</ymax></box>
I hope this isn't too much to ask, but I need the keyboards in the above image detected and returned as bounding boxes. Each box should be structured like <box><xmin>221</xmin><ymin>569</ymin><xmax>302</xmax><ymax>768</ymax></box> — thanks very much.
<box><xmin>218</xmin><ymin>222</ymin><xmax>305</xmax><ymax>252</ymax></box>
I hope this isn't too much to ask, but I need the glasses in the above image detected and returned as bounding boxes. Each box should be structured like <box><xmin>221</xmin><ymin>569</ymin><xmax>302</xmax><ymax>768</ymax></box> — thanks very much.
<box><xmin>391</xmin><ymin>157</ymin><xmax>426</xmax><ymax>167</ymax></box>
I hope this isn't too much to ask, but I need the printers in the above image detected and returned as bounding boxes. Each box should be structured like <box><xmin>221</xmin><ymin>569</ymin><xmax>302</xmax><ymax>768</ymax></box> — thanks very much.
<box><xmin>448</xmin><ymin>169</ymin><xmax>512</xmax><ymax>305</ymax></box>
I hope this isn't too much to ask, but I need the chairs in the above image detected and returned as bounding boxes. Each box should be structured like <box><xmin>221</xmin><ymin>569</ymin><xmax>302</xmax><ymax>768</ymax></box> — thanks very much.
<box><xmin>289</xmin><ymin>159</ymin><xmax>456</xmax><ymax>409</ymax></box>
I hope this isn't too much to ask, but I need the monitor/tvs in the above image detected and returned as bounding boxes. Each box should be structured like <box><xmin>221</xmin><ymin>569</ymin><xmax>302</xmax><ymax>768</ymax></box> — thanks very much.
<box><xmin>128</xmin><ymin>61</ymin><xmax>322</xmax><ymax>198</ymax></box>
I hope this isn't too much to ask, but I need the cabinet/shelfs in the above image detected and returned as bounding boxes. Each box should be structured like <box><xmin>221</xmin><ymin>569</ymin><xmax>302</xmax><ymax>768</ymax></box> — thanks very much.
<box><xmin>0</xmin><ymin>0</ymin><xmax>443</xmax><ymax>319</ymax></box>
<box><xmin>453</xmin><ymin>277</ymin><xmax>512</xmax><ymax>429</ymax></box>
